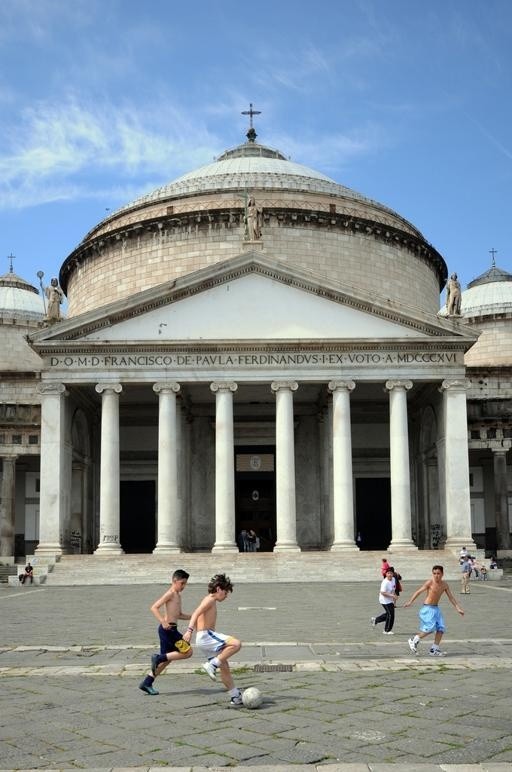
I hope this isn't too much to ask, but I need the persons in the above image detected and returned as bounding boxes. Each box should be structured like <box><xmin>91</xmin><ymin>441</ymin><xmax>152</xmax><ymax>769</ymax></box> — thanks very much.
<box><xmin>489</xmin><ymin>556</ymin><xmax>497</xmax><ymax>568</ymax></box>
<box><xmin>469</xmin><ymin>558</ymin><xmax>479</xmax><ymax>577</ymax></box>
<box><xmin>393</xmin><ymin>571</ymin><xmax>403</xmax><ymax>608</ymax></box>
<box><xmin>239</xmin><ymin>528</ymin><xmax>262</xmax><ymax>552</ymax></box>
<box><xmin>459</xmin><ymin>554</ymin><xmax>471</xmax><ymax>594</ymax></box>
<box><xmin>241</xmin><ymin>196</ymin><xmax>264</xmax><ymax>243</ymax></box>
<box><xmin>444</xmin><ymin>272</ymin><xmax>463</xmax><ymax>315</ymax></box>
<box><xmin>371</xmin><ymin>566</ymin><xmax>399</xmax><ymax>635</ymax></box>
<box><xmin>40</xmin><ymin>277</ymin><xmax>64</xmax><ymax>322</ymax></box>
<box><xmin>459</xmin><ymin>546</ymin><xmax>468</xmax><ymax>564</ymax></box>
<box><xmin>401</xmin><ymin>564</ymin><xmax>465</xmax><ymax>656</ymax></box>
<box><xmin>21</xmin><ymin>562</ymin><xmax>34</xmax><ymax>584</ymax></box>
<box><xmin>380</xmin><ymin>558</ymin><xmax>390</xmax><ymax>578</ymax></box>
<box><xmin>182</xmin><ymin>571</ymin><xmax>244</xmax><ymax>705</ymax></box>
<box><xmin>137</xmin><ymin>569</ymin><xmax>193</xmax><ymax>695</ymax></box>
<box><xmin>480</xmin><ymin>565</ymin><xmax>487</xmax><ymax>580</ymax></box>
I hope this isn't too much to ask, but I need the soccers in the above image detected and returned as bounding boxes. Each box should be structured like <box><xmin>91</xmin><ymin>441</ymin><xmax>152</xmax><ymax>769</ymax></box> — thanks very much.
<box><xmin>241</xmin><ymin>687</ymin><xmax>263</xmax><ymax>709</ymax></box>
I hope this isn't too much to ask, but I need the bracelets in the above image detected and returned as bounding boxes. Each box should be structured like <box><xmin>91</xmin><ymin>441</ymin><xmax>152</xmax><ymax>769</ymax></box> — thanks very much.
<box><xmin>187</xmin><ymin>626</ymin><xmax>193</xmax><ymax>632</ymax></box>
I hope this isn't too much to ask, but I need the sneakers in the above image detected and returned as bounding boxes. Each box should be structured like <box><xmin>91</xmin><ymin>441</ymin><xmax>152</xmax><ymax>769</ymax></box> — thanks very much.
<box><xmin>231</xmin><ymin>691</ymin><xmax>244</xmax><ymax>705</ymax></box>
<box><xmin>428</xmin><ymin>648</ymin><xmax>448</xmax><ymax>657</ymax></box>
<box><xmin>383</xmin><ymin>631</ymin><xmax>395</xmax><ymax>635</ymax></box>
<box><xmin>152</xmin><ymin>654</ymin><xmax>160</xmax><ymax>676</ymax></box>
<box><xmin>371</xmin><ymin>617</ymin><xmax>377</xmax><ymax>630</ymax></box>
<box><xmin>202</xmin><ymin>662</ymin><xmax>217</xmax><ymax>682</ymax></box>
<box><xmin>139</xmin><ymin>682</ymin><xmax>159</xmax><ymax>696</ymax></box>
<box><xmin>408</xmin><ymin>637</ymin><xmax>419</xmax><ymax>653</ymax></box>
